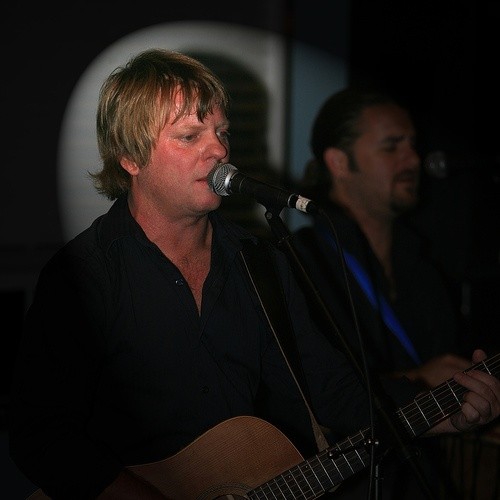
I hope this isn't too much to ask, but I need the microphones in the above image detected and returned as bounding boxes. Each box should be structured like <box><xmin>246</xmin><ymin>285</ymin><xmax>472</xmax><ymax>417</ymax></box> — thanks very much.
<box><xmin>207</xmin><ymin>163</ymin><xmax>317</xmax><ymax>217</ymax></box>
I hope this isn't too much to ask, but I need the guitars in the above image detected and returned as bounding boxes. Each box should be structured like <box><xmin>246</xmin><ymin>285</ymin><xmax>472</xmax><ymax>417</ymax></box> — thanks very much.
<box><xmin>95</xmin><ymin>351</ymin><xmax>500</xmax><ymax>500</ymax></box>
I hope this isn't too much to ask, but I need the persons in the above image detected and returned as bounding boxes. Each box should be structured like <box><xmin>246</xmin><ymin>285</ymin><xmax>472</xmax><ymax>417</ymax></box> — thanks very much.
<box><xmin>290</xmin><ymin>82</ymin><xmax>500</xmax><ymax>500</ymax></box>
<box><xmin>0</xmin><ymin>47</ymin><xmax>500</xmax><ymax>500</ymax></box>
